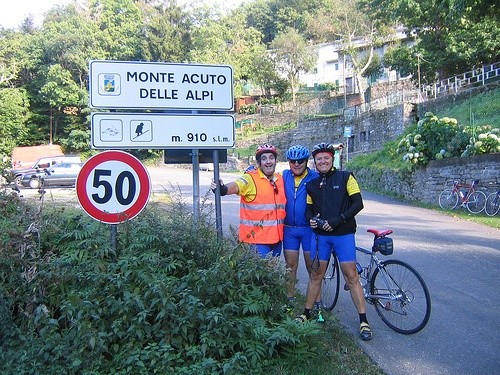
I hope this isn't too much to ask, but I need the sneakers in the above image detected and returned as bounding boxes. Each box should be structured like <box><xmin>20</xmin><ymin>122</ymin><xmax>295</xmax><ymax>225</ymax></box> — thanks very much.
<box><xmin>314</xmin><ymin>306</ymin><xmax>326</xmax><ymax>324</ymax></box>
<box><xmin>293</xmin><ymin>314</ymin><xmax>311</xmax><ymax>323</ymax></box>
<box><xmin>360</xmin><ymin>322</ymin><xmax>373</xmax><ymax>341</ymax></box>
<box><xmin>285</xmin><ymin>297</ymin><xmax>296</xmax><ymax>316</ymax></box>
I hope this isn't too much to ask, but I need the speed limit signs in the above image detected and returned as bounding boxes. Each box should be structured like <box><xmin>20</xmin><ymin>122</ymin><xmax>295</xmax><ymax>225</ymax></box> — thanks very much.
<box><xmin>75</xmin><ymin>150</ymin><xmax>152</xmax><ymax>225</ymax></box>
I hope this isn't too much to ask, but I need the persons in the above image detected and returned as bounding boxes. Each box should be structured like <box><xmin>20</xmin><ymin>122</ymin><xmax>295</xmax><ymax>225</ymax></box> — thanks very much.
<box><xmin>210</xmin><ymin>144</ymin><xmax>286</xmax><ymax>262</ymax></box>
<box><xmin>295</xmin><ymin>143</ymin><xmax>372</xmax><ymax>341</ymax></box>
<box><xmin>243</xmin><ymin>144</ymin><xmax>326</xmax><ymax>322</ymax></box>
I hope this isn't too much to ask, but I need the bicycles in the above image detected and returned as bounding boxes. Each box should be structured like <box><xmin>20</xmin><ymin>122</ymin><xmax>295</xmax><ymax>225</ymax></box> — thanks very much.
<box><xmin>320</xmin><ymin>228</ymin><xmax>432</xmax><ymax>335</ymax></box>
<box><xmin>438</xmin><ymin>179</ymin><xmax>488</xmax><ymax>214</ymax></box>
<box><xmin>484</xmin><ymin>181</ymin><xmax>500</xmax><ymax>217</ymax></box>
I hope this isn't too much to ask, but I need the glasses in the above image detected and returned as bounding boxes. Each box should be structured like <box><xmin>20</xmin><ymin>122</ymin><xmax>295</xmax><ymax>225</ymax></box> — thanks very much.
<box><xmin>290</xmin><ymin>159</ymin><xmax>304</xmax><ymax>164</ymax></box>
<box><xmin>270</xmin><ymin>179</ymin><xmax>279</xmax><ymax>195</ymax></box>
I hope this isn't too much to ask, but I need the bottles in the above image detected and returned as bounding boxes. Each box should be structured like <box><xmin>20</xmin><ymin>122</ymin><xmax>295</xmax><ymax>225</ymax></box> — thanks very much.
<box><xmin>354</xmin><ymin>261</ymin><xmax>363</xmax><ymax>274</ymax></box>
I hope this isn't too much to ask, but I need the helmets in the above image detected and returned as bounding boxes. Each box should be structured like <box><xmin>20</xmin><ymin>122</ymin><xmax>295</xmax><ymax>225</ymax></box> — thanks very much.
<box><xmin>285</xmin><ymin>145</ymin><xmax>310</xmax><ymax>161</ymax></box>
<box><xmin>255</xmin><ymin>143</ymin><xmax>277</xmax><ymax>157</ymax></box>
<box><xmin>312</xmin><ymin>142</ymin><xmax>335</xmax><ymax>156</ymax></box>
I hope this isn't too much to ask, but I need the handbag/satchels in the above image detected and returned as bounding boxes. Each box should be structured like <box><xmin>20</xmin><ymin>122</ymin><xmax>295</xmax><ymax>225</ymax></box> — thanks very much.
<box><xmin>375</xmin><ymin>237</ymin><xmax>394</xmax><ymax>255</ymax></box>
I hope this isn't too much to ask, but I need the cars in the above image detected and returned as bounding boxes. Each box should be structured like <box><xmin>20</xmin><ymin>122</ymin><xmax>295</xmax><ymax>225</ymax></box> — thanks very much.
<box><xmin>3</xmin><ymin>155</ymin><xmax>86</xmax><ymax>189</ymax></box>
